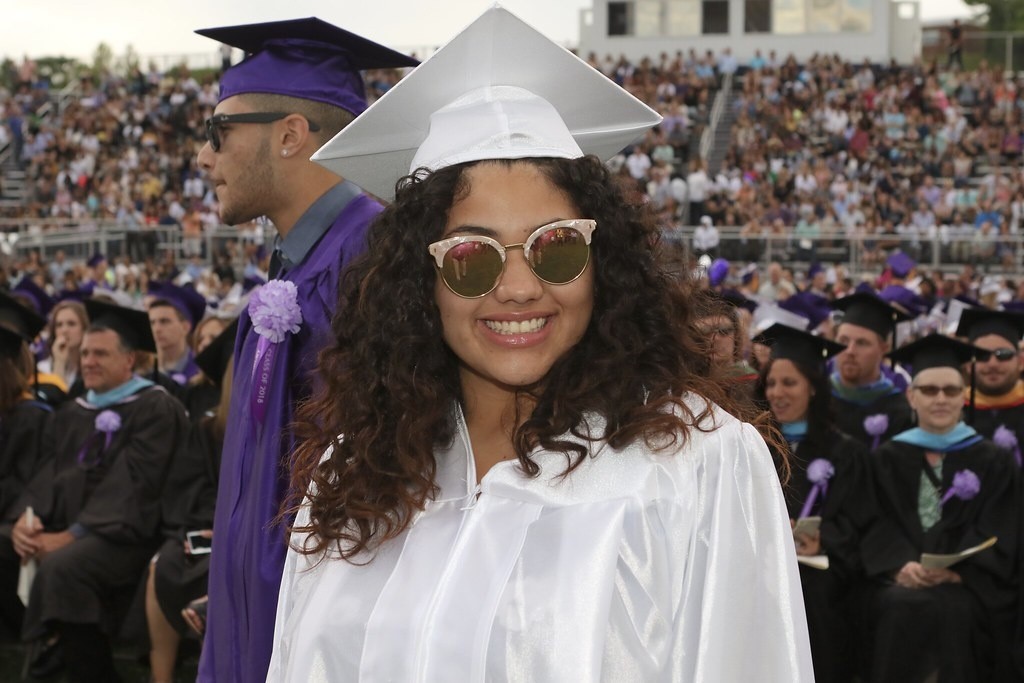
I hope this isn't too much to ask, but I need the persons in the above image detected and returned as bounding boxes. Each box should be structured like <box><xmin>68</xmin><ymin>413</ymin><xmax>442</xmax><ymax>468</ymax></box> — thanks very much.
<box><xmin>0</xmin><ymin>16</ymin><xmax>443</xmax><ymax>683</ymax></box>
<box><xmin>555</xmin><ymin>46</ymin><xmax>1024</xmax><ymax>683</ymax></box>
<box><xmin>193</xmin><ymin>2</ymin><xmax>817</xmax><ymax>683</ymax></box>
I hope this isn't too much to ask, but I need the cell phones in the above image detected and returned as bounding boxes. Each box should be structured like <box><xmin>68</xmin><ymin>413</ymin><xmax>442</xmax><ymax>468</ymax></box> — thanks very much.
<box><xmin>186</xmin><ymin>530</ymin><xmax>212</xmax><ymax>554</ymax></box>
<box><xmin>25</xmin><ymin>506</ymin><xmax>35</xmax><ymax>534</ymax></box>
<box><xmin>793</xmin><ymin>516</ymin><xmax>823</xmax><ymax>546</ymax></box>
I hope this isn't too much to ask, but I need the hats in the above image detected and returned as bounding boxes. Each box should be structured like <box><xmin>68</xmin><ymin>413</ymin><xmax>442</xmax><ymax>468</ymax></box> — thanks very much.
<box><xmin>257</xmin><ymin>245</ymin><xmax>269</xmax><ymax>258</ymax></box>
<box><xmin>145</xmin><ymin>280</ymin><xmax>206</xmax><ymax>334</ymax></box>
<box><xmin>308</xmin><ymin>4</ymin><xmax>665</xmax><ymax>203</ymax></box>
<box><xmin>956</xmin><ymin>308</ymin><xmax>1024</xmax><ymax>344</ymax></box>
<box><xmin>88</xmin><ymin>253</ymin><xmax>105</xmax><ymax>268</ymax></box>
<box><xmin>0</xmin><ymin>292</ymin><xmax>49</xmax><ymax>401</ymax></box>
<box><xmin>707</xmin><ymin>253</ymin><xmax>919</xmax><ymax>331</ymax></box>
<box><xmin>830</xmin><ymin>291</ymin><xmax>912</xmax><ymax>372</ymax></box>
<box><xmin>883</xmin><ymin>333</ymin><xmax>993</xmax><ymax>426</ymax></box>
<box><xmin>193</xmin><ymin>17</ymin><xmax>422</xmax><ymax>118</ymax></box>
<box><xmin>83</xmin><ymin>296</ymin><xmax>161</xmax><ymax>386</ymax></box>
<box><xmin>751</xmin><ymin>322</ymin><xmax>847</xmax><ymax>369</ymax></box>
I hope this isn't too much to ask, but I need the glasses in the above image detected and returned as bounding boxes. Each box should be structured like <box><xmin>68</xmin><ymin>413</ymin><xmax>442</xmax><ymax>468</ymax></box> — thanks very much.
<box><xmin>974</xmin><ymin>348</ymin><xmax>1018</xmax><ymax>363</ymax></box>
<box><xmin>206</xmin><ymin>111</ymin><xmax>320</xmax><ymax>152</ymax></box>
<box><xmin>913</xmin><ymin>385</ymin><xmax>963</xmax><ymax>397</ymax></box>
<box><xmin>426</xmin><ymin>219</ymin><xmax>598</xmax><ymax>299</ymax></box>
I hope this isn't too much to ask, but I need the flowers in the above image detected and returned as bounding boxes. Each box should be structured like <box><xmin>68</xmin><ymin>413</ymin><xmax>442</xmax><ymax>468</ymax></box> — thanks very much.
<box><xmin>807</xmin><ymin>458</ymin><xmax>836</xmax><ymax>486</ymax></box>
<box><xmin>248</xmin><ymin>279</ymin><xmax>304</xmax><ymax>345</ymax></box>
<box><xmin>95</xmin><ymin>408</ymin><xmax>123</xmax><ymax>433</ymax></box>
<box><xmin>865</xmin><ymin>414</ymin><xmax>889</xmax><ymax>436</ymax></box>
<box><xmin>942</xmin><ymin>469</ymin><xmax>981</xmax><ymax>502</ymax></box>
<box><xmin>994</xmin><ymin>424</ymin><xmax>1017</xmax><ymax>451</ymax></box>
